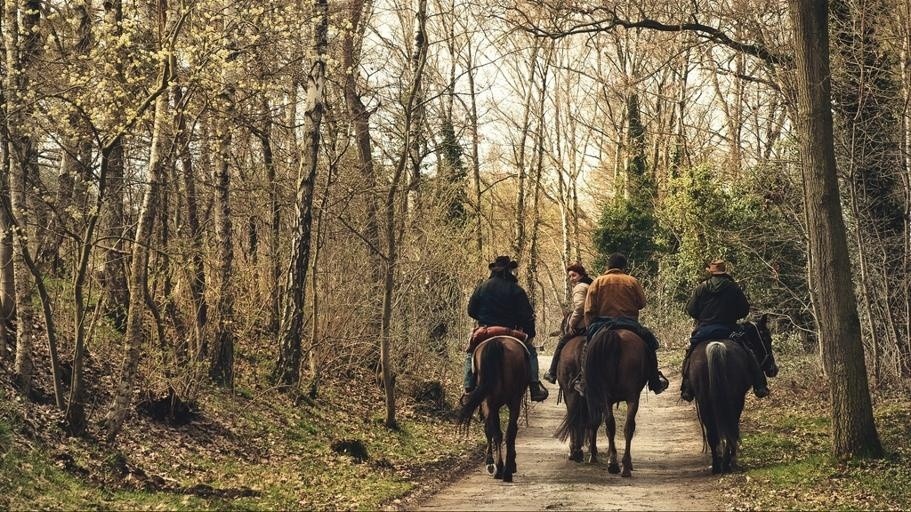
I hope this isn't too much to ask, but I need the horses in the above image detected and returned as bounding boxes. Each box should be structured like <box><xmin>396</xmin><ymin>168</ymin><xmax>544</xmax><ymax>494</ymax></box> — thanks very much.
<box><xmin>688</xmin><ymin>313</ymin><xmax>780</xmax><ymax>476</ymax></box>
<box><xmin>440</xmin><ymin>337</ymin><xmax>530</xmax><ymax>482</ymax></box>
<box><xmin>550</xmin><ymin>311</ymin><xmax>603</xmax><ymax>464</ymax></box>
<box><xmin>586</xmin><ymin>328</ymin><xmax>649</xmax><ymax>477</ymax></box>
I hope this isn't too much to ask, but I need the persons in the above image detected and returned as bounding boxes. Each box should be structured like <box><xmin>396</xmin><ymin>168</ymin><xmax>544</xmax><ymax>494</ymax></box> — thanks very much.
<box><xmin>681</xmin><ymin>258</ymin><xmax>771</xmax><ymax>402</ymax></box>
<box><xmin>573</xmin><ymin>252</ymin><xmax>667</xmax><ymax>397</ymax></box>
<box><xmin>462</xmin><ymin>254</ymin><xmax>547</xmax><ymax>405</ymax></box>
<box><xmin>543</xmin><ymin>264</ymin><xmax>595</xmax><ymax>383</ymax></box>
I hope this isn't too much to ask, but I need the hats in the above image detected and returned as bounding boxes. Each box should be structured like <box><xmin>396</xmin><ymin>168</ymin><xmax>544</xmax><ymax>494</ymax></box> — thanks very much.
<box><xmin>705</xmin><ymin>260</ymin><xmax>729</xmax><ymax>275</ymax></box>
<box><xmin>488</xmin><ymin>255</ymin><xmax>517</xmax><ymax>272</ymax></box>
<box><xmin>607</xmin><ymin>253</ymin><xmax>627</xmax><ymax>269</ymax></box>
<box><xmin>567</xmin><ymin>261</ymin><xmax>587</xmax><ymax>276</ymax></box>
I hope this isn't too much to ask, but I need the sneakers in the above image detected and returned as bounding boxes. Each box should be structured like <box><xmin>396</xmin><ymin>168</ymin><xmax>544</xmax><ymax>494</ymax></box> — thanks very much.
<box><xmin>754</xmin><ymin>385</ymin><xmax>771</xmax><ymax>399</ymax></box>
<box><xmin>650</xmin><ymin>373</ymin><xmax>669</xmax><ymax>395</ymax></box>
<box><xmin>543</xmin><ymin>367</ymin><xmax>556</xmax><ymax>385</ymax></box>
<box><xmin>680</xmin><ymin>389</ymin><xmax>695</xmax><ymax>403</ymax></box>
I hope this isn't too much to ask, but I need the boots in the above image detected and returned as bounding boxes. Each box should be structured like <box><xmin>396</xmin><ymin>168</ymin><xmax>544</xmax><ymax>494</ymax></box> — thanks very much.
<box><xmin>529</xmin><ymin>381</ymin><xmax>549</xmax><ymax>401</ymax></box>
<box><xmin>460</xmin><ymin>393</ymin><xmax>473</xmax><ymax>406</ymax></box>
